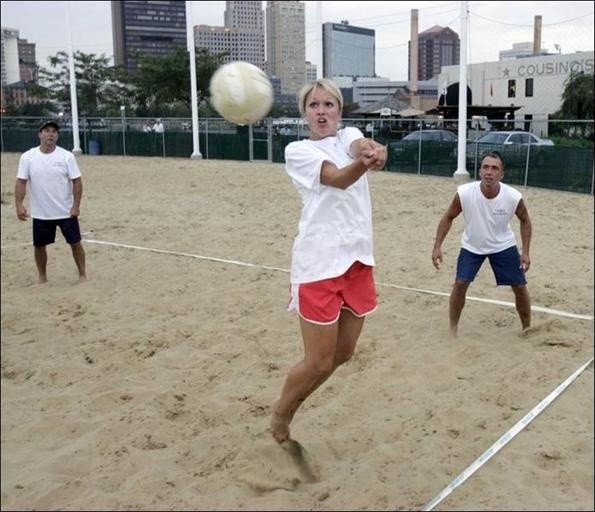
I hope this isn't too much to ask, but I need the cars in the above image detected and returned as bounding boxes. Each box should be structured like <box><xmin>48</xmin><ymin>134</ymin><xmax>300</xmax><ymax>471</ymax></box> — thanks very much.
<box><xmin>450</xmin><ymin>130</ymin><xmax>555</xmax><ymax>168</ymax></box>
<box><xmin>77</xmin><ymin>118</ymin><xmax>106</xmax><ymax>128</ymax></box>
<box><xmin>386</xmin><ymin>128</ymin><xmax>458</xmax><ymax>164</ymax></box>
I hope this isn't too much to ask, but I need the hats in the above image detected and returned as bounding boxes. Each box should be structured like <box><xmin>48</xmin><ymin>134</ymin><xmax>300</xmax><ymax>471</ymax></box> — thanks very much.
<box><xmin>37</xmin><ymin>117</ymin><xmax>59</xmax><ymax>133</ymax></box>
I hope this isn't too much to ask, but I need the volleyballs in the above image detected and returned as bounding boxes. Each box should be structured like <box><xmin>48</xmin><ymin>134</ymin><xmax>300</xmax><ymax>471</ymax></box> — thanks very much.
<box><xmin>210</xmin><ymin>59</ymin><xmax>276</xmax><ymax>127</ymax></box>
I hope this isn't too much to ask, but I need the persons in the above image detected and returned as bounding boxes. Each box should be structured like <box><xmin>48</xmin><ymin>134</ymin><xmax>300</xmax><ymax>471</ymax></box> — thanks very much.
<box><xmin>152</xmin><ymin>119</ymin><xmax>164</xmax><ymax>134</ymax></box>
<box><xmin>266</xmin><ymin>79</ymin><xmax>390</xmax><ymax>447</ymax></box>
<box><xmin>432</xmin><ymin>152</ymin><xmax>534</xmax><ymax>335</ymax></box>
<box><xmin>12</xmin><ymin>119</ymin><xmax>88</xmax><ymax>284</ymax></box>
